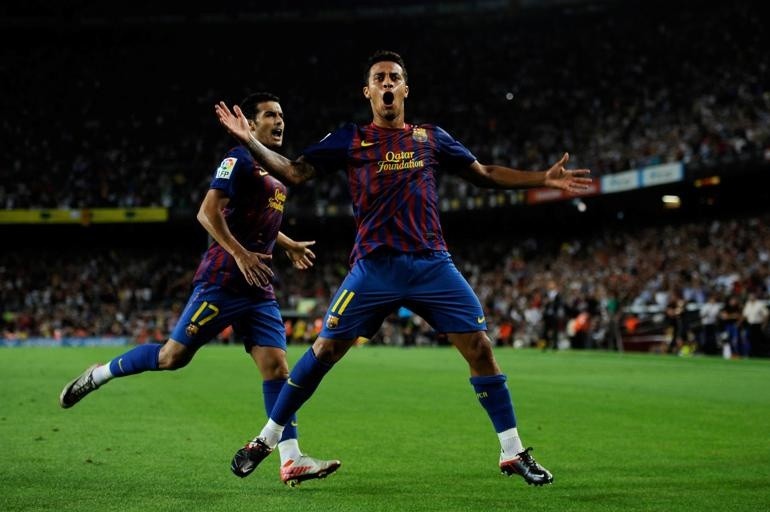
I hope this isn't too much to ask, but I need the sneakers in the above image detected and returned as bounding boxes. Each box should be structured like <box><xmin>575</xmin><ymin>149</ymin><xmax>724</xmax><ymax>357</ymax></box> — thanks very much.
<box><xmin>281</xmin><ymin>453</ymin><xmax>341</xmax><ymax>486</ymax></box>
<box><xmin>58</xmin><ymin>362</ymin><xmax>108</xmax><ymax>409</ymax></box>
<box><xmin>230</xmin><ymin>436</ymin><xmax>277</xmax><ymax>478</ymax></box>
<box><xmin>499</xmin><ymin>447</ymin><xmax>553</xmax><ymax>488</ymax></box>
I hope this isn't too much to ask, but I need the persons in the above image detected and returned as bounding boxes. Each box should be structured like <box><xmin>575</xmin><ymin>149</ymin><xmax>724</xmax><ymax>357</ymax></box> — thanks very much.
<box><xmin>211</xmin><ymin>49</ymin><xmax>597</xmax><ymax>490</ymax></box>
<box><xmin>56</xmin><ymin>88</ymin><xmax>345</xmax><ymax>490</ymax></box>
<box><xmin>0</xmin><ymin>2</ymin><xmax>770</xmax><ymax>359</ymax></box>
